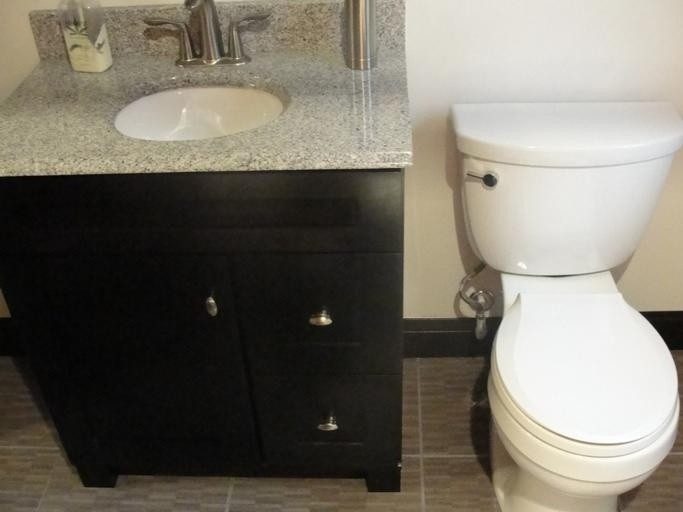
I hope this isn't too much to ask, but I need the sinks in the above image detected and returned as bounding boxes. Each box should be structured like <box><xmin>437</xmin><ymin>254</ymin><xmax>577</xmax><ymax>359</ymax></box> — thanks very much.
<box><xmin>114</xmin><ymin>81</ymin><xmax>288</xmax><ymax>143</ymax></box>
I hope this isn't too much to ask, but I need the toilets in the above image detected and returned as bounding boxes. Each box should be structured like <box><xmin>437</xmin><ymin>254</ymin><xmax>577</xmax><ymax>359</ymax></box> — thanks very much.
<box><xmin>452</xmin><ymin>101</ymin><xmax>682</xmax><ymax>512</ymax></box>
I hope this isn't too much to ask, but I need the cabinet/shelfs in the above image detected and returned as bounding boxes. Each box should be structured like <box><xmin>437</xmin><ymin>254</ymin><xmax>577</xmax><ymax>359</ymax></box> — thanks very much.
<box><xmin>0</xmin><ymin>169</ymin><xmax>405</xmax><ymax>491</ymax></box>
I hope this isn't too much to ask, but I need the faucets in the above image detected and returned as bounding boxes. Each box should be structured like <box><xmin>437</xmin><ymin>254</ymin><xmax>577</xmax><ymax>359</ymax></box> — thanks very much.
<box><xmin>185</xmin><ymin>1</ymin><xmax>224</xmax><ymax>63</ymax></box>
